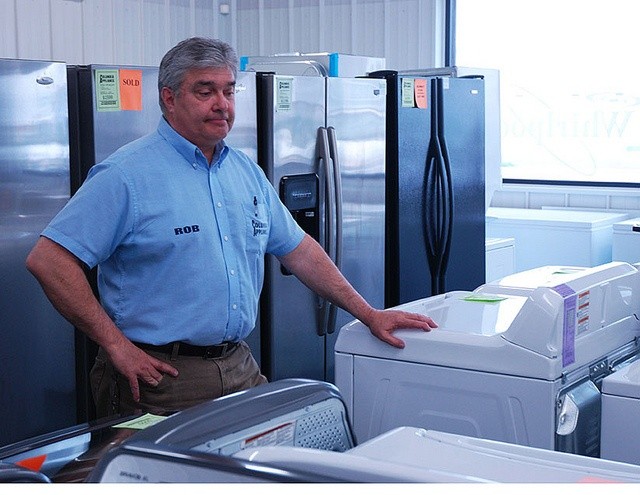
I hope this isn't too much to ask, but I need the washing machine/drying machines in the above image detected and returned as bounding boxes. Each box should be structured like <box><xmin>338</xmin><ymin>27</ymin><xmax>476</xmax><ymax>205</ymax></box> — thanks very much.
<box><xmin>333</xmin><ymin>258</ymin><xmax>639</xmax><ymax>459</ymax></box>
<box><xmin>474</xmin><ymin>262</ymin><xmax>640</xmax><ymax>295</ymax></box>
<box><xmin>238</xmin><ymin>424</ymin><xmax>639</xmax><ymax>482</ymax></box>
<box><xmin>600</xmin><ymin>358</ymin><xmax>639</xmax><ymax>464</ymax></box>
<box><xmin>0</xmin><ymin>372</ymin><xmax>358</xmax><ymax>484</ymax></box>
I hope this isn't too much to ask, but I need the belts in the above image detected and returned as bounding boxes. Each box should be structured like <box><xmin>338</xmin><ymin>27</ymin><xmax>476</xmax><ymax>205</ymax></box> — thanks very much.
<box><xmin>130</xmin><ymin>338</ymin><xmax>244</xmax><ymax>358</ymax></box>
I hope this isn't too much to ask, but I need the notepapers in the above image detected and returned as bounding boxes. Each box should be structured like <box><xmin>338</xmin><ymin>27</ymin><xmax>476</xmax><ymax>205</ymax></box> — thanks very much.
<box><xmin>414</xmin><ymin>78</ymin><xmax>427</xmax><ymax>108</ymax></box>
<box><xmin>458</xmin><ymin>295</ymin><xmax>507</xmax><ymax>301</ymax></box>
<box><xmin>113</xmin><ymin>411</ymin><xmax>169</xmax><ymax>429</ymax></box>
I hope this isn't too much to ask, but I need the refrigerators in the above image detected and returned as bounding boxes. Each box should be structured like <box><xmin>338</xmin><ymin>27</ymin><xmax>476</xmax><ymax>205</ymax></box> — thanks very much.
<box><xmin>71</xmin><ymin>61</ymin><xmax>263</xmax><ymax>373</ymax></box>
<box><xmin>372</xmin><ymin>66</ymin><xmax>487</xmax><ymax>295</ymax></box>
<box><xmin>265</xmin><ymin>71</ymin><xmax>391</xmax><ymax>385</ymax></box>
<box><xmin>0</xmin><ymin>54</ymin><xmax>95</xmax><ymax>452</ymax></box>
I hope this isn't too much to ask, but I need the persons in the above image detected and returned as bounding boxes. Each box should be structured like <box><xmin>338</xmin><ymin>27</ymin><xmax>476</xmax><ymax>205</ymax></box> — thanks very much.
<box><xmin>25</xmin><ymin>37</ymin><xmax>439</xmax><ymax>417</ymax></box>
